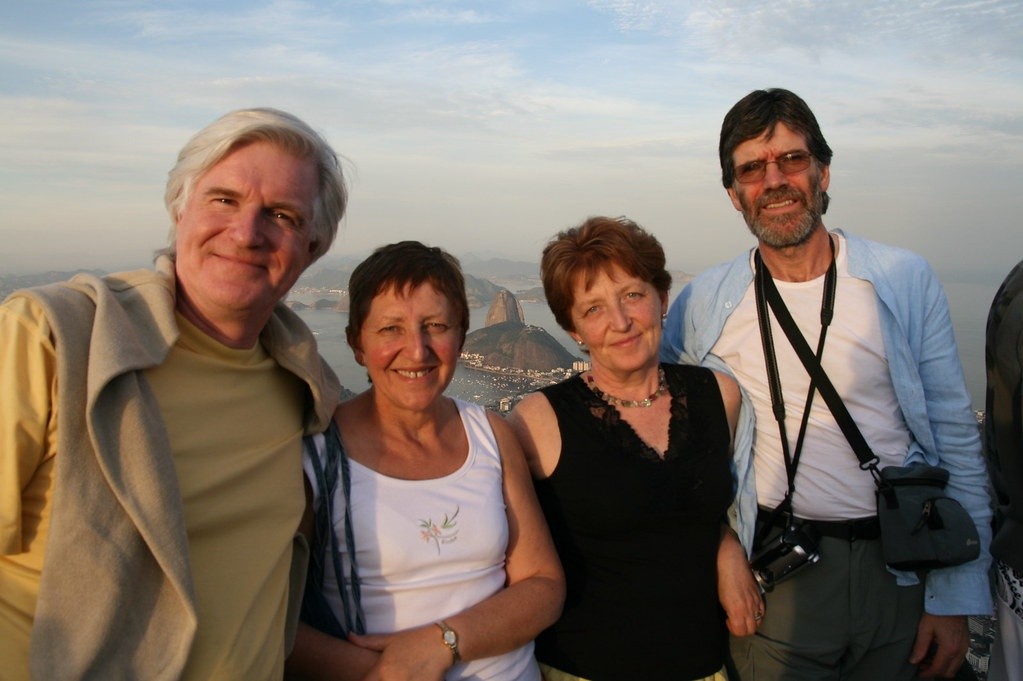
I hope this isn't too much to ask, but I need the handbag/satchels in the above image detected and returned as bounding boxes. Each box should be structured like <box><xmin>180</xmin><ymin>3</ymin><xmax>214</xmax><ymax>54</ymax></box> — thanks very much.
<box><xmin>872</xmin><ymin>465</ymin><xmax>981</xmax><ymax>573</ymax></box>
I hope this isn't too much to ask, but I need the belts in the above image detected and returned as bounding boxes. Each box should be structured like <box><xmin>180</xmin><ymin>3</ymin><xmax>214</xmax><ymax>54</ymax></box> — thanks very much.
<box><xmin>812</xmin><ymin>520</ymin><xmax>882</xmax><ymax>542</ymax></box>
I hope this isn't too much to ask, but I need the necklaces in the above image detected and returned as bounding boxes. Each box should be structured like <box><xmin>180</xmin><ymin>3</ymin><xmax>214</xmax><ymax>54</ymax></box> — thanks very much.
<box><xmin>588</xmin><ymin>370</ymin><xmax>665</xmax><ymax>408</ymax></box>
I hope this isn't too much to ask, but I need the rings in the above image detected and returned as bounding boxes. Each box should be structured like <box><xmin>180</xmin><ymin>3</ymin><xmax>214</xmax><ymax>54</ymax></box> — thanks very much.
<box><xmin>753</xmin><ymin>610</ymin><xmax>763</xmax><ymax>621</ymax></box>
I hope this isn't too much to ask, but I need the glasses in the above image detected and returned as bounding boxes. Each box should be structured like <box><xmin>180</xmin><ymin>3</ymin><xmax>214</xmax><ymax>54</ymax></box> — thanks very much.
<box><xmin>731</xmin><ymin>149</ymin><xmax>813</xmax><ymax>185</ymax></box>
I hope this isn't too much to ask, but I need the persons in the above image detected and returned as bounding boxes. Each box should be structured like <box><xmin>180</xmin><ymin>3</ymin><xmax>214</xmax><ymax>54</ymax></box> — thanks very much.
<box><xmin>504</xmin><ymin>217</ymin><xmax>767</xmax><ymax>681</ymax></box>
<box><xmin>977</xmin><ymin>256</ymin><xmax>1023</xmax><ymax>681</ymax></box>
<box><xmin>660</xmin><ymin>87</ymin><xmax>990</xmax><ymax>681</ymax></box>
<box><xmin>0</xmin><ymin>106</ymin><xmax>342</xmax><ymax>681</ymax></box>
<box><xmin>300</xmin><ymin>240</ymin><xmax>565</xmax><ymax>681</ymax></box>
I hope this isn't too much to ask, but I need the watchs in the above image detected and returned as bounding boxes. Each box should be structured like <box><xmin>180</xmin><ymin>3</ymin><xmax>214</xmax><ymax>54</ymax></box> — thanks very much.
<box><xmin>433</xmin><ymin>619</ymin><xmax>460</xmax><ymax>660</ymax></box>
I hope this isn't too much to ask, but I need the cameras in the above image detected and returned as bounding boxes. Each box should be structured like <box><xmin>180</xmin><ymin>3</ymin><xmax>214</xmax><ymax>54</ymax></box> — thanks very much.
<box><xmin>749</xmin><ymin>524</ymin><xmax>823</xmax><ymax>588</ymax></box>
